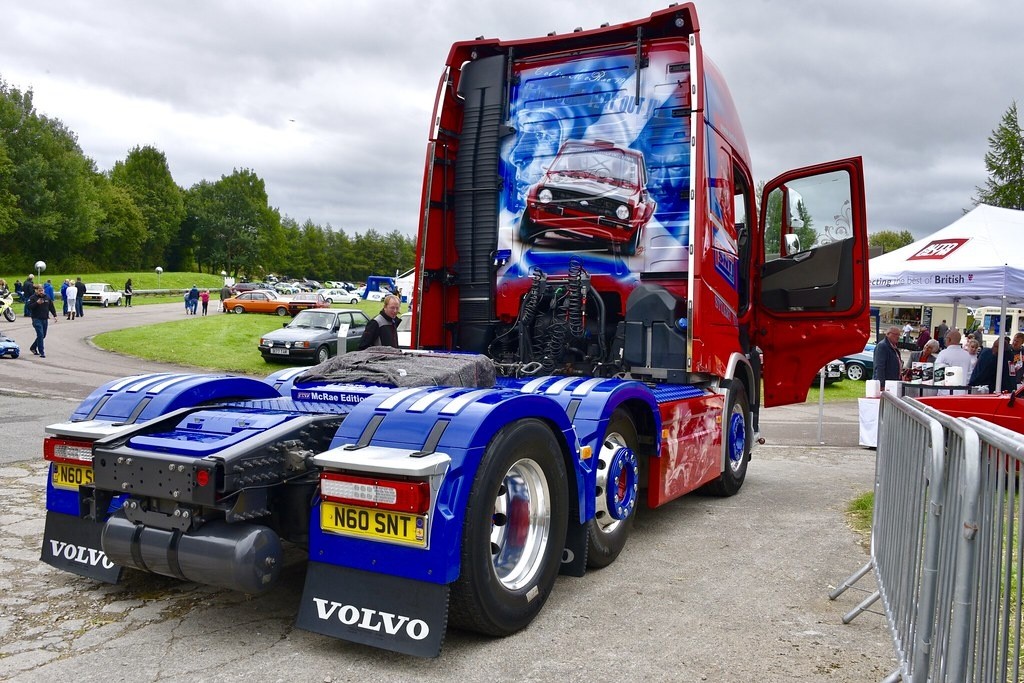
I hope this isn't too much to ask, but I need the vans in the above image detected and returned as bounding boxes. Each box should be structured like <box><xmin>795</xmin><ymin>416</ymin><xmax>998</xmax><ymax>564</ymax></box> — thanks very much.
<box><xmin>971</xmin><ymin>306</ymin><xmax>1024</xmax><ymax>349</ymax></box>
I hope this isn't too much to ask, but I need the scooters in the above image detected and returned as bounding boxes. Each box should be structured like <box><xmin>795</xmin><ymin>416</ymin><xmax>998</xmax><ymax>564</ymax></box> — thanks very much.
<box><xmin>0</xmin><ymin>284</ymin><xmax>17</xmax><ymax>322</ymax></box>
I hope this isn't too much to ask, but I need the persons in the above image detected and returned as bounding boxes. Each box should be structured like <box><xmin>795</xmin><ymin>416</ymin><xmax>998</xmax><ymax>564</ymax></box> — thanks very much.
<box><xmin>42</xmin><ymin>279</ymin><xmax>55</xmax><ymax>302</ymax></box>
<box><xmin>124</xmin><ymin>278</ymin><xmax>133</xmax><ymax>307</ymax></box>
<box><xmin>872</xmin><ymin>326</ymin><xmax>904</xmax><ymax>392</ymax></box>
<box><xmin>25</xmin><ymin>284</ymin><xmax>58</xmax><ymax>358</ymax></box>
<box><xmin>220</xmin><ymin>285</ymin><xmax>235</xmax><ymax>314</ymax></box>
<box><xmin>359</xmin><ymin>294</ymin><xmax>403</xmax><ymax>353</ymax></box>
<box><xmin>903</xmin><ymin>320</ymin><xmax>1024</xmax><ymax>404</ymax></box>
<box><xmin>61</xmin><ymin>277</ymin><xmax>86</xmax><ymax>320</ymax></box>
<box><xmin>183</xmin><ymin>284</ymin><xmax>210</xmax><ymax>317</ymax></box>
<box><xmin>14</xmin><ymin>274</ymin><xmax>58</xmax><ymax>318</ymax></box>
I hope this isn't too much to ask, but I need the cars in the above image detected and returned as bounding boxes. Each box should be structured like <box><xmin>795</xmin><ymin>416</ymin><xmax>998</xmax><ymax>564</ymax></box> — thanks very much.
<box><xmin>231</xmin><ymin>277</ymin><xmax>367</xmax><ymax>304</ymax></box>
<box><xmin>838</xmin><ymin>350</ymin><xmax>876</xmax><ymax>381</ymax></box>
<box><xmin>242</xmin><ymin>290</ymin><xmax>293</xmax><ymax>302</ymax></box>
<box><xmin>813</xmin><ymin>360</ymin><xmax>847</xmax><ymax>386</ymax></box>
<box><xmin>0</xmin><ymin>335</ymin><xmax>21</xmax><ymax>359</ymax></box>
<box><xmin>288</xmin><ymin>292</ymin><xmax>331</xmax><ymax>317</ymax></box>
<box><xmin>397</xmin><ymin>313</ymin><xmax>413</xmax><ymax>349</ymax></box>
<box><xmin>259</xmin><ymin>308</ymin><xmax>372</xmax><ymax>365</ymax></box>
<box><xmin>83</xmin><ymin>283</ymin><xmax>123</xmax><ymax>307</ymax></box>
<box><xmin>223</xmin><ymin>291</ymin><xmax>293</xmax><ymax>316</ymax></box>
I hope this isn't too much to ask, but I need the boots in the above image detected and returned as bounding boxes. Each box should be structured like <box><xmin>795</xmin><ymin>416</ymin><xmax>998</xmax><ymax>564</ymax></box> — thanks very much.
<box><xmin>72</xmin><ymin>312</ymin><xmax>75</xmax><ymax>320</ymax></box>
<box><xmin>67</xmin><ymin>312</ymin><xmax>70</xmax><ymax>320</ymax></box>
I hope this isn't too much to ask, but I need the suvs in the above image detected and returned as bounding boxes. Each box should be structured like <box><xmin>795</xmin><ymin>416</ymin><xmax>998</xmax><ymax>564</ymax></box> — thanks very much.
<box><xmin>518</xmin><ymin>139</ymin><xmax>658</xmax><ymax>257</ymax></box>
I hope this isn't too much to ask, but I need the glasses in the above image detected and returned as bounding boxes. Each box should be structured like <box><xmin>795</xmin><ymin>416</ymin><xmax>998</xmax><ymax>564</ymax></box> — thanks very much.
<box><xmin>890</xmin><ymin>332</ymin><xmax>901</xmax><ymax>337</ymax></box>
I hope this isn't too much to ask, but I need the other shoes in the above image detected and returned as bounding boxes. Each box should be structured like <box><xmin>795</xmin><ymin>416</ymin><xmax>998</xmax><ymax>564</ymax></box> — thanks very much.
<box><xmin>40</xmin><ymin>353</ymin><xmax>45</xmax><ymax>358</ymax></box>
<box><xmin>30</xmin><ymin>349</ymin><xmax>39</xmax><ymax>355</ymax></box>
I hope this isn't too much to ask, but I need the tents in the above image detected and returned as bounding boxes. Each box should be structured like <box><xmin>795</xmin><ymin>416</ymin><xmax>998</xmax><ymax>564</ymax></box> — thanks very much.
<box><xmin>816</xmin><ymin>203</ymin><xmax>1023</xmax><ymax>441</ymax></box>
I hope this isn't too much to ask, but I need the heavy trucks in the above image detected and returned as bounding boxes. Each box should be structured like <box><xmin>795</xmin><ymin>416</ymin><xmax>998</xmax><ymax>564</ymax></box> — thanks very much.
<box><xmin>40</xmin><ymin>1</ymin><xmax>874</xmax><ymax>661</ymax></box>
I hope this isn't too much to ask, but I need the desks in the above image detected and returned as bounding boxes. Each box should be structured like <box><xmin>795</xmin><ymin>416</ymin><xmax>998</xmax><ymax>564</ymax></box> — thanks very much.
<box><xmin>902</xmin><ymin>382</ymin><xmax>971</xmax><ymax>397</ymax></box>
<box><xmin>857</xmin><ymin>397</ymin><xmax>880</xmax><ymax>448</ymax></box>
